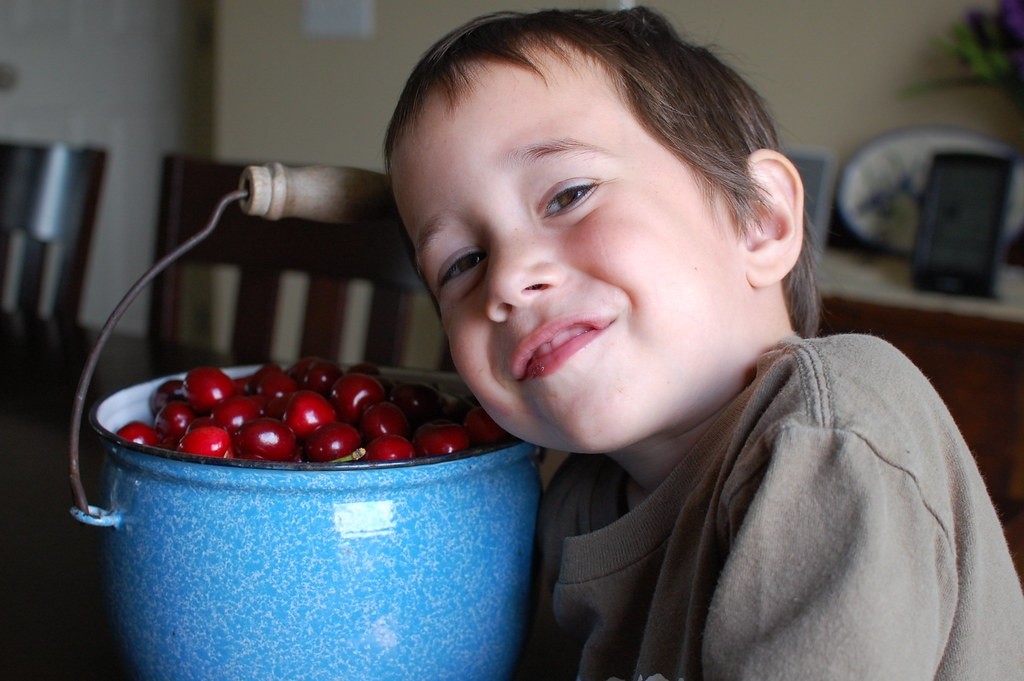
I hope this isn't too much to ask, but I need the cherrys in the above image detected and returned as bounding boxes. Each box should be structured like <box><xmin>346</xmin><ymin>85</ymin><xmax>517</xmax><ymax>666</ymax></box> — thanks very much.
<box><xmin>115</xmin><ymin>354</ymin><xmax>506</xmax><ymax>464</ymax></box>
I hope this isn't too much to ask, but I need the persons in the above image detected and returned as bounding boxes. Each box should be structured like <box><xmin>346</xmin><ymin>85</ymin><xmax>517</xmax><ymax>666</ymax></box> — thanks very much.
<box><xmin>383</xmin><ymin>8</ymin><xmax>1024</xmax><ymax>681</ymax></box>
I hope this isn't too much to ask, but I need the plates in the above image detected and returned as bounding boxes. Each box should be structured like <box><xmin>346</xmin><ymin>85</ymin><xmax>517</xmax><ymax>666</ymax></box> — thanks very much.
<box><xmin>839</xmin><ymin>128</ymin><xmax>1024</xmax><ymax>257</ymax></box>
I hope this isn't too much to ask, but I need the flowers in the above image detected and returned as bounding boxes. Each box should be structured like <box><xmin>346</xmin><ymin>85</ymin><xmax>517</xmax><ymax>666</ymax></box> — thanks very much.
<box><xmin>918</xmin><ymin>0</ymin><xmax>1023</xmax><ymax>114</ymax></box>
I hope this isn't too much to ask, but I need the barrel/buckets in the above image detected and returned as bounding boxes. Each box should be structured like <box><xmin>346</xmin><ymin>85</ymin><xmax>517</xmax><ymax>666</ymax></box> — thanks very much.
<box><xmin>69</xmin><ymin>164</ymin><xmax>542</xmax><ymax>681</ymax></box>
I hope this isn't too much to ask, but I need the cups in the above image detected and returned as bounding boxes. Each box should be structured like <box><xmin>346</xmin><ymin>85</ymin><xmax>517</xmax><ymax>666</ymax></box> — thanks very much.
<box><xmin>912</xmin><ymin>152</ymin><xmax>1014</xmax><ymax>296</ymax></box>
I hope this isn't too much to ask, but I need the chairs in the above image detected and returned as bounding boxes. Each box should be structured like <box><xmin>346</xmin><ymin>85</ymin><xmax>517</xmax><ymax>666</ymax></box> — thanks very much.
<box><xmin>1</xmin><ymin>141</ymin><xmax>110</xmax><ymax>317</ymax></box>
<box><xmin>145</xmin><ymin>151</ymin><xmax>460</xmax><ymax>371</ymax></box>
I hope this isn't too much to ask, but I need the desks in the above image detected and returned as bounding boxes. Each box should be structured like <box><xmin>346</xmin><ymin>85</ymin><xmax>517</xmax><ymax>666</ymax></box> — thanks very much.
<box><xmin>809</xmin><ymin>246</ymin><xmax>1024</xmax><ymax>587</ymax></box>
<box><xmin>0</xmin><ymin>309</ymin><xmax>307</xmax><ymax>681</ymax></box>
